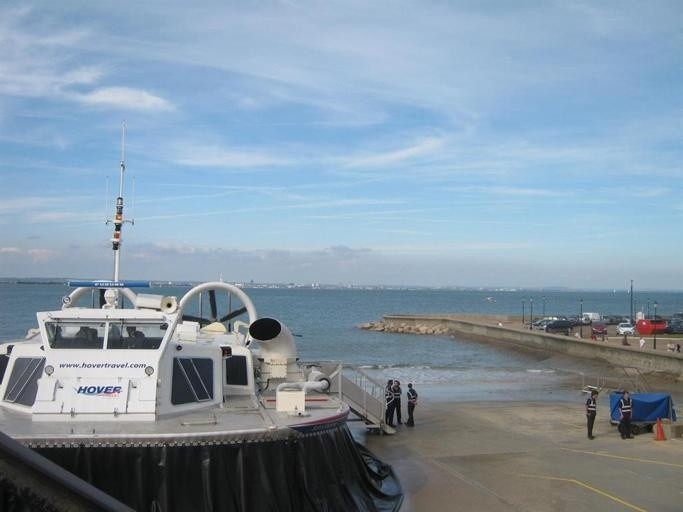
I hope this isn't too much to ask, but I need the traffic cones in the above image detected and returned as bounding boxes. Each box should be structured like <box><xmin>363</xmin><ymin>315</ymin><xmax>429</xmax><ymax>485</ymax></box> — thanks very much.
<box><xmin>656</xmin><ymin>416</ymin><xmax>666</xmax><ymax>440</ymax></box>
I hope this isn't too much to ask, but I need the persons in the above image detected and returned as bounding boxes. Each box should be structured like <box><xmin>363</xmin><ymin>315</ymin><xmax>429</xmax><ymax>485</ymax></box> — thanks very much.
<box><xmin>591</xmin><ymin>331</ymin><xmax>598</xmax><ymax>341</ymax></box>
<box><xmin>391</xmin><ymin>380</ymin><xmax>404</xmax><ymax>425</ymax></box>
<box><xmin>600</xmin><ymin>332</ymin><xmax>608</xmax><ymax>343</ymax></box>
<box><xmin>574</xmin><ymin>331</ymin><xmax>581</xmax><ymax>339</ymax></box>
<box><xmin>385</xmin><ymin>380</ymin><xmax>396</xmax><ymax>428</ymax></box>
<box><xmin>403</xmin><ymin>383</ymin><xmax>417</xmax><ymax>427</ymax></box>
<box><xmin>564</xmin><ymin>329</ymin><xmax>569</xmax><ymax>336</ymax></box>
<box><xmin>585</xmin><ymin>390</ymin><xmax>599</xmax><ymax>440</ymax></box>
<box><xmin>639</xmin><ymin>336</ymin><xmax>646</xmax><ymax>348</ymax></box>
<box><xmin>622</xmin><ymin>335</ymin><xmax>631</xmax><ymax>346</ymax></box>
<box><xmin>617</xmin><ymin>391</ymin><xmax>634</xmax><ymax>439</ymax></box>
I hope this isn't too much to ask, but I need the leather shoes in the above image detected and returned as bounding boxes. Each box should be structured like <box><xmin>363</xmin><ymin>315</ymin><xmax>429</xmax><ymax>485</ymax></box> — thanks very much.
<box><xmin>622</xmin><ymin>435</ymin><xmax>634</xmax><ymax>439</ymax></box>
<box><xmin>389</xmin><ymin>421</ymin><xmax>413</xmax><ymax>428</ymax></box>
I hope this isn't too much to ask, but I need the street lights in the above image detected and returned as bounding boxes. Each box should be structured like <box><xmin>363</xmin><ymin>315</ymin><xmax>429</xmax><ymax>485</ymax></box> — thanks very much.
<box><xmin>633</xmin><ymin>296</ymin><xmax>637</xmax><ymax>322</ymax></box>
<box><xmin>653</xmin><ymin>301</ymin><xmax>657</xmax><ymax>350</ymax></box>
<box><xmin>580</xmin><ymin>299</ymin><xmax>583</xmax><ymax>337</ymax></box>
<box><xmin>521</xmin><ymin>295</ymin><xmax>546</xmax><ymax>330</ymax></box>
<box><xmin>646</xmin><ymin>298</ymin><xmax>651</xmax><ymax>319</ymax></box>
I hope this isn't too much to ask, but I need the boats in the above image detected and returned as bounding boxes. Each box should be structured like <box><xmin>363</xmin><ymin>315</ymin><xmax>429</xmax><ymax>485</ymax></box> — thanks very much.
<box><xmin>3</xmin><ymin>120</ymin><xmax>403</xmax><ymax>512</ymax></box>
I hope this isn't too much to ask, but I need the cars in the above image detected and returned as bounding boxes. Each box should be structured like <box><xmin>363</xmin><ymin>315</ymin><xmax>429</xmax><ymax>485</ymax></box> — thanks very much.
<box><xmin>529</xmin><ymin>312</ymin><xmax>682</xmax><ymax>341</ymax></box>
